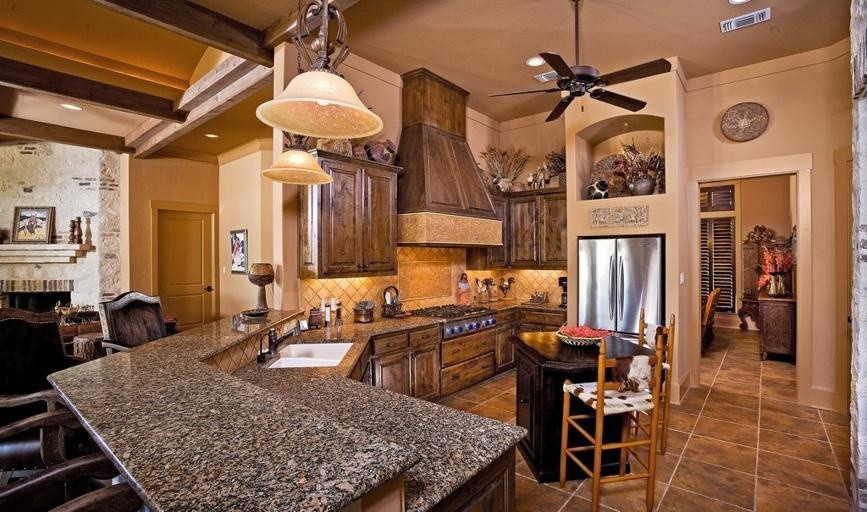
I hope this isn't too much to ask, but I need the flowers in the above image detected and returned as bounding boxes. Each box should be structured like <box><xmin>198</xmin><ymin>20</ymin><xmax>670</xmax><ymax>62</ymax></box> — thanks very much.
<box><xmin>760</xmin><ymin>242</ymin><xmax>795</xmax><ymax>275</ymax></box>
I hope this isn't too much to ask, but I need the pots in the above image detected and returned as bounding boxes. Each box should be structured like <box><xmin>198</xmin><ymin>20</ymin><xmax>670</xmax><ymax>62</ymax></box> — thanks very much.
<box><xmin>352</xmin><ymin>301</ymin><xmax>374</xmax><ymax>324</ymax></box>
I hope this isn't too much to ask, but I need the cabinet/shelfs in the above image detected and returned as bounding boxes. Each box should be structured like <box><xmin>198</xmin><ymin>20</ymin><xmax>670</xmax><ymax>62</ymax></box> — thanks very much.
<box><xmin>508</xmin><ymin>188</ymin><xmax>569</xmax><ymax>272</ymax></box>
<box><xmin>466</xmin><ymin>186</ymin><xmax>508</xmax><ymax>270</ymax></box>
<box><xmin>295</xmin><ymin>147</ymin><xmax>406</xmax><ymax>278</ymax></box>
<box><xmin>442</xmin><ymin>310</ymin><xmax>571</xmax><ymax>395</ymax></box>
<box><xmin>398</xmin><ymin>66</ymin><xmax>470</xmax><ymax>139</ymax></box>
<box><xmin>512</xmin><ymin>326</ymin><xmax>630</xmax><ymax>480</ymax></box>
<box><xmin>758</xmin><ymin>286</ymin><xmax>797</xmax><ymax>364</ymax></box>
<box><xmin>371</xmin><ymin>326</ymin><xmax>442</xmax><ymax>406</ymax></box>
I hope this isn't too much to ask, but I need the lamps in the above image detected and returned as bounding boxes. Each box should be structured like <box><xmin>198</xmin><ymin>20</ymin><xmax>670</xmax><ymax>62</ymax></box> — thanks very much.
<box><xmin>255</xmin><ymin>0</ymin><xmax>384</xmax><ymax>186</ymax></box>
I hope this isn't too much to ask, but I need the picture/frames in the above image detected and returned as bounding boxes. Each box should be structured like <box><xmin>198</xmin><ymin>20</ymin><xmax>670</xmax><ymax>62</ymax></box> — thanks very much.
<box><xmin>230</xmin><ymin>228</ymin><xmax>249</xmax><ymax>274</ymax></box>
<box><xmin>11</xmin><ymin>204</ymin><xmax>55</xmax><ymax>243</ymax></box>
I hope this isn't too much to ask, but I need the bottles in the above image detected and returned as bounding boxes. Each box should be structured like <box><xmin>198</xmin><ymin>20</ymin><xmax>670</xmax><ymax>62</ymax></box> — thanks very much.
<box><xmin>268</xmin><ymin>329</ymin><xmax>277</xmax><ymax>354</ymax></box>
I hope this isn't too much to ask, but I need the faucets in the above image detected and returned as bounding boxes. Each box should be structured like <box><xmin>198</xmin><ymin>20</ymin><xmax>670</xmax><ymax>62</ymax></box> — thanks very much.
<box><xmin>258</xmin><ymin>334</ymin><xmax>276</xmax><ymax>354</ymax></box>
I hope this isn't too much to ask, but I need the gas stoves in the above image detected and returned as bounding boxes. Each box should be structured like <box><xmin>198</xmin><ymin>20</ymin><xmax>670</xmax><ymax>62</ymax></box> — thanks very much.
<box><xmin>412</xmin><ymin>303</ymin><xmax>488</xmax><ymax>318</ymax></box>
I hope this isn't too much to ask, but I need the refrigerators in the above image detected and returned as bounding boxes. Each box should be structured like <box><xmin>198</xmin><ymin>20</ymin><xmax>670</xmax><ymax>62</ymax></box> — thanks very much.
<box><xmin>578</xmin><ymin>237</ymin><xmax>662</xmax><ymax>396</ymax></box>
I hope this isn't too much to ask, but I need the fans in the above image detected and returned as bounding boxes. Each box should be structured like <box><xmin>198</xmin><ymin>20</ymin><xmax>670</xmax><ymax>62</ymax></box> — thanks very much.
<box><xmin>487</xmin><ymin>0</ymin><xmax>670</xmax><ymax>123</ymax></box>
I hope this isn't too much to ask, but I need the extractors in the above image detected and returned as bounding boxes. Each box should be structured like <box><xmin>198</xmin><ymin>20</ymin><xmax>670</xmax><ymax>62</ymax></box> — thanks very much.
<box><xmin>398</xmin><ymin>68</ymin><xmax>507</xmax><ymax>248</ymax></box>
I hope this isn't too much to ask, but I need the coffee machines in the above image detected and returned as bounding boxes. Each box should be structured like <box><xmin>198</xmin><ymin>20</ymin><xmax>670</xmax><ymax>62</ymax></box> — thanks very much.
<box><xmin>558</xmin><ymin>277</ymin><xmax>566</xmax><ymax>308</ymax></box>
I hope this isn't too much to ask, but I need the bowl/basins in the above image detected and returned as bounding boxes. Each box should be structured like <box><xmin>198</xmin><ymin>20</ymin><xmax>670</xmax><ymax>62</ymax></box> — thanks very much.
<box><xmin>555</xmin><ymin>331</ymin><xmax>609</xmax><ymax>347</ymax></box>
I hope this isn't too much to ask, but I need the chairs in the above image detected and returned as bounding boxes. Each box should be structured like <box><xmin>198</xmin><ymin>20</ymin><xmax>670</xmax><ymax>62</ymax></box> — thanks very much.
<box><xmin>701</xmin><ymin>287</ymin><xmax>720</xmax><ymax>347</ymax></box>
<box><xmin>561</xmin><ymin>308</ymin><xmax>675</xmax><ymax>511</ymax></box>
<box><xmin>0</xmin><ymin>291</ymin><xmax>173</xmax><ymax>511</ymax></box>
